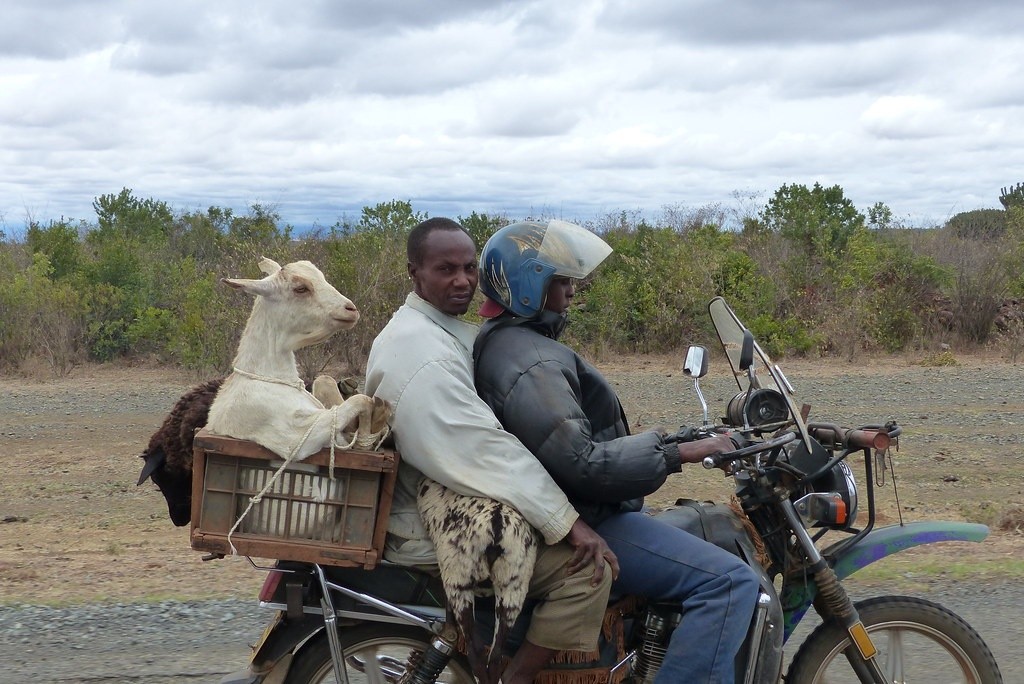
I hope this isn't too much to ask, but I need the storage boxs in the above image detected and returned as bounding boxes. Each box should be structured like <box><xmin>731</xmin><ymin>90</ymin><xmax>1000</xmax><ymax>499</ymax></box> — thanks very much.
<box><xmin>189</xmin><ymin>426</ymin><xmax>400</xmax><ymax>572</ymax></box>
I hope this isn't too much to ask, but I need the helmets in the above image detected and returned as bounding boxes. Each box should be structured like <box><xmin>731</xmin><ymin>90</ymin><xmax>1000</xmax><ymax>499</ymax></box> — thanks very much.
<box><xmin>477</xmin><ymin>221</ymin><xmax>584</xmax><ymax>319</ymax></box>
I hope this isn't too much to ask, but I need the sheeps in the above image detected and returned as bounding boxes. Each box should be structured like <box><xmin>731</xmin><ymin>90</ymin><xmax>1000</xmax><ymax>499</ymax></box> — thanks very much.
<box><xmin>137</xmin><ymin>256</ymin><xmax>393</xmax><ymax>527</ymax></box>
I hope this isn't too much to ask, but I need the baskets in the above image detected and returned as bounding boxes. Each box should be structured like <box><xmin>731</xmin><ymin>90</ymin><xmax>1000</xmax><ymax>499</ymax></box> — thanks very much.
<box><xmin>200</xmin><ymin>453</ymin><xmax>379</xmax><ymax>551</ymax></box>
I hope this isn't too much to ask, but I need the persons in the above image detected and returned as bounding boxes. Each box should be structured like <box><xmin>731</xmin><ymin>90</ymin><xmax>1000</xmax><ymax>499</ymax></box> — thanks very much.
<box><xmin>473</xmin><ymin>217</ymin><xmax>760</xmax><ymax>684</ymax></box>
<box><xmin>364</xmin><ymin>217</ymin><xmax>622</xmax><ymax>684</ymax></box>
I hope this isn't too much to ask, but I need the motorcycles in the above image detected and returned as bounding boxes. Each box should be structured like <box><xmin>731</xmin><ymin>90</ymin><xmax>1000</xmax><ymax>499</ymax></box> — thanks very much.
<box><xmin>219</xmin><ymin>296</ymin><xmax>1003</xmax><ymax>684</ymax></box>
